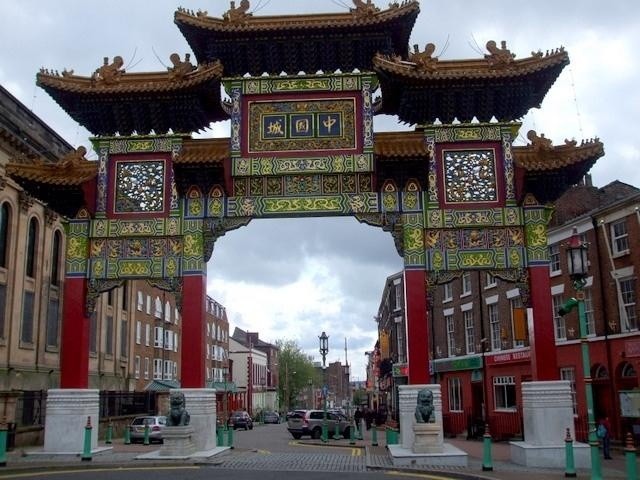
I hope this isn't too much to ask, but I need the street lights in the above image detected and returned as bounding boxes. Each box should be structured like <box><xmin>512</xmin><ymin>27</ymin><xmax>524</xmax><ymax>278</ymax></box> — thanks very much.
<box><xmin>560</xmin><ymin>225</ymin><xmax>603</xmax><ymax>480</ymax></box>
<box><xmin>222</xmin><ymin>366</ymin><xmax>229</xmax><ymax>421</ymax></box>
<box><xmin>317</xmin><ymin>331</ymin><xmax>330</xmax><ymax>411</ymax></box>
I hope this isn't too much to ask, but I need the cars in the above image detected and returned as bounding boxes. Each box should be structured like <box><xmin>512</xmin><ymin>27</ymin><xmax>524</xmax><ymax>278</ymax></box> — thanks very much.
<box><xmin>227</xmin><ymin>410</ymin><xmax>254</xmax><ymax>431</ymax></box>
<box><xmin>128</xmin><ymin>415</ymin><xmax>166</xmax><ymax>443</ymax></box>
<box><xmin>264</xmin><ymin>411</ymin><xmax>281</xmax><ymax>425</ymax></box>
<box><xmin>286</xmin><ymin>409</ymin><xmax>355</xmax><ymax>440</ymax></box>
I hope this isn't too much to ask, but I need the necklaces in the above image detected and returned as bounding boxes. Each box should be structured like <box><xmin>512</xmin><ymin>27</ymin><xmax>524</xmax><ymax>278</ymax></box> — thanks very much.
<box><xmin>354</xmin><ymin>407</ymin><xmax>362</xmax><ymax>431</ymax></box>
<box><xmin>362</xmin><ymin>405</ymin><xmax>371</xmax><ymax>430</ymax></box>
<box><xmin>597</xmin><ymin>413</ymin><xmax>615</xmax><ymax>460</ymax></box>
